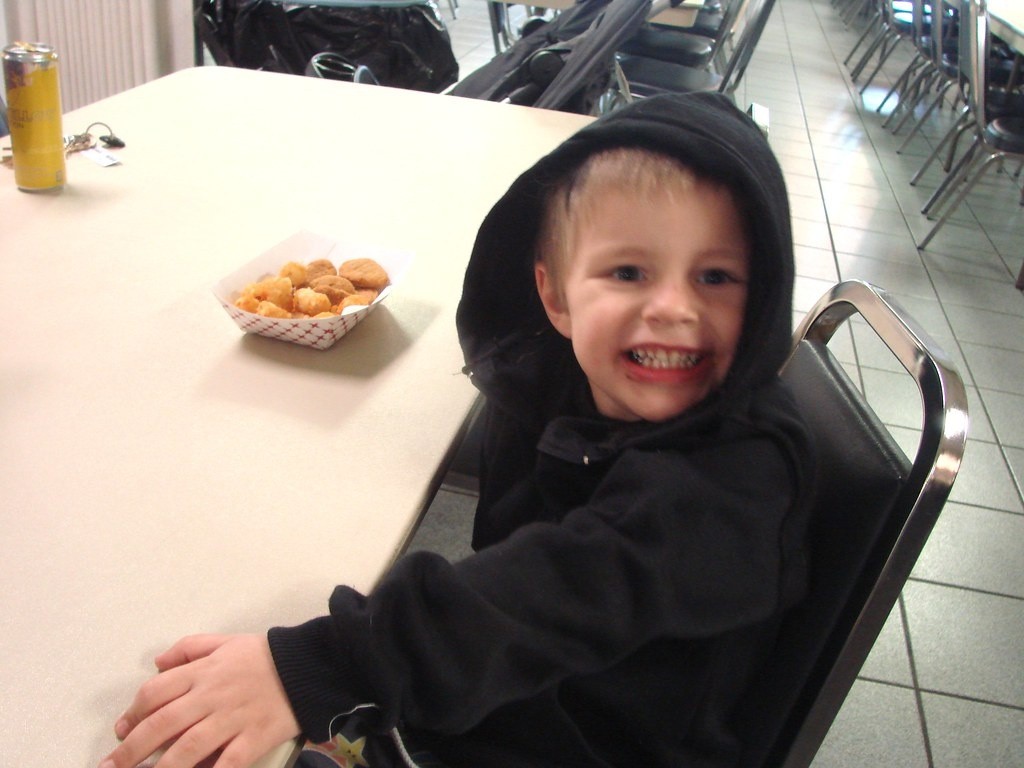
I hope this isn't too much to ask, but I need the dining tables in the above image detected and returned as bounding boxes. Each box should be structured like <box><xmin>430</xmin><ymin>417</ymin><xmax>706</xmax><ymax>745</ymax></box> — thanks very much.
<box><xmin>0</xmin><ymin>66</ymin><xmax>599</xmax><ymax>768</ymax></box>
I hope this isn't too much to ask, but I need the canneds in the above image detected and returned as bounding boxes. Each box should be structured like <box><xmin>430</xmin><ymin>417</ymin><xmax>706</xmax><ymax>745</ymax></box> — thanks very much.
<box><xmin>0</xmin><ymin>42</ymin><xmax>67</xmax><ymax>192</ymax></box>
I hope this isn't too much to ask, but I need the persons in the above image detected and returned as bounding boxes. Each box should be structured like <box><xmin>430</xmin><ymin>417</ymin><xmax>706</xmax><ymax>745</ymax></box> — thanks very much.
<box><xmin>93</xmin><ymin>89</ymin><xmax>816</xmax><ymax>768</ymax></box>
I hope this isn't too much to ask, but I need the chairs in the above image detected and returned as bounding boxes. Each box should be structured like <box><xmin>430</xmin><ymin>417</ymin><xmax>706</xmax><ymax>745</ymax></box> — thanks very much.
<box><xmin>691</xmin><ymin>279</ymin><xmax>967</xmax><ymax>768</ymax></box>
<box><xmin>311</xmin><ymin>0</ymin><xmax>778</xmax><ymax>152</ymax></box>
<box><xmin>843</xmin><ymin>0</ymin><xmax>1024</xmax><ymax>289</ymax></box>
